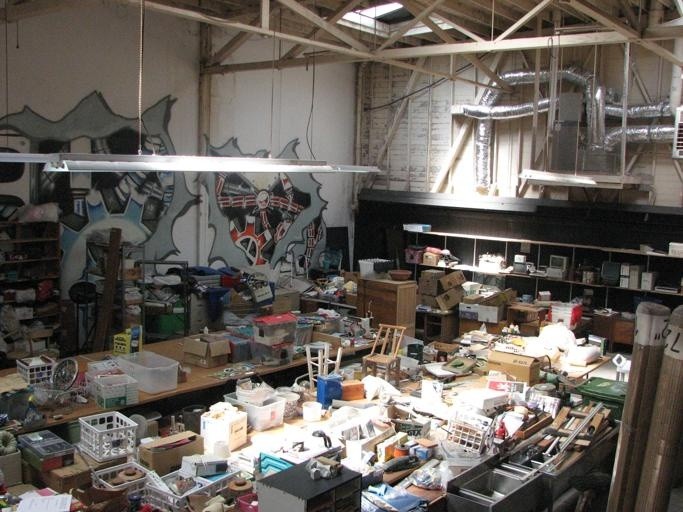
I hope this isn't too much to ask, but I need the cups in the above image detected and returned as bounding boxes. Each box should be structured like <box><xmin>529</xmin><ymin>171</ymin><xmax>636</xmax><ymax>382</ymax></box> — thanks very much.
<box><xmin>301</xmin><ymin>400</ymin><xmax>321</xmax><ymax>421</ymax></box>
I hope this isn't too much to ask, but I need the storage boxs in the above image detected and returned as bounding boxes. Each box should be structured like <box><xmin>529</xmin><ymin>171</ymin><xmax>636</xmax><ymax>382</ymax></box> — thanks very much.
<box><xmin>354</xmin><ymin>266</ymin><xmax>546</xmax><ymax>337</ymax></box>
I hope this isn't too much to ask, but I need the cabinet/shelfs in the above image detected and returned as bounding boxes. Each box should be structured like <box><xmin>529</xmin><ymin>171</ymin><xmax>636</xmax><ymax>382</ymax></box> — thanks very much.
<box><xmin>85</xmin><ymin>241</ymin><xmax>145</xmax><ymax>338</ymax></box>
<box><xmin>404</xmin><ymin>227</ymin><xmax>681</xmax><ymax>315</ymax></box>
<box><xmin>1</xmin><ymin>217</ymin><xmax>61</xmax><ymax>320</ymax></box>
<box><xmin>141</xmin><ymin>258</ymin><xmax>191</xmax><ymax>336</ymax></box>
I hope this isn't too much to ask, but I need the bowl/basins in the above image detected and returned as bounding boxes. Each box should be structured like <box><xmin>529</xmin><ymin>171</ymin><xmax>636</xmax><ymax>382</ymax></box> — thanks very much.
<box><xmin>389</xmin><ymin>269</ymin><xmax>412</xmax><ymax>281</ymax></box>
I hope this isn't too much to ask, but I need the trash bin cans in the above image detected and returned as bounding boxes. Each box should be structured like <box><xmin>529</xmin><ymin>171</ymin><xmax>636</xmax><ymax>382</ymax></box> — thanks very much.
<box><xmin>575</xmin><ymin>376</ymin><xmax>629</xmax><ymax>421</ymax></box>
